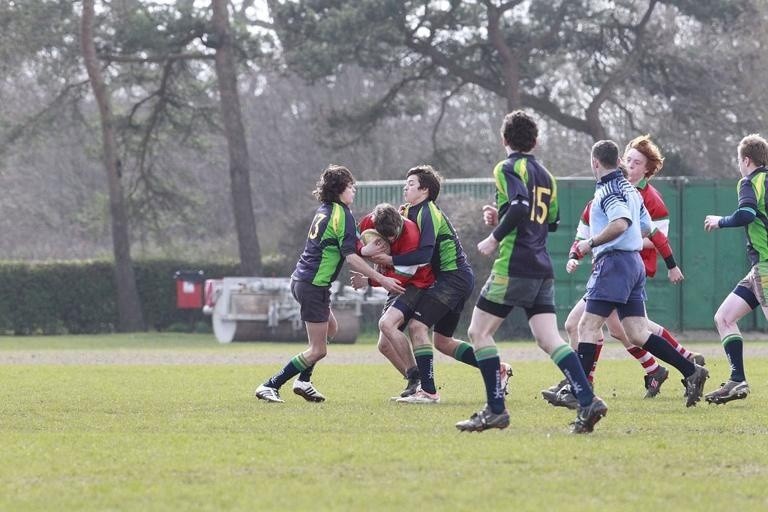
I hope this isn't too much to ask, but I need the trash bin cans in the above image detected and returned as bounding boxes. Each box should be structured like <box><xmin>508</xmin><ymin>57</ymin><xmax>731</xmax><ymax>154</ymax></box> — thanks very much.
<box><xmin>176</xmin><ymin>270</ymin><xmax>204</xmax><ymax>310</ymax></box>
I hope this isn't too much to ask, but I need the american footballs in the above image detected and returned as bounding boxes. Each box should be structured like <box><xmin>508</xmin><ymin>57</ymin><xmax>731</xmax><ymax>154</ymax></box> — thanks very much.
<box><xmin>360</xmin><ymin>229</ymin><xmax>391</xmax><ymax>255</ymax></box>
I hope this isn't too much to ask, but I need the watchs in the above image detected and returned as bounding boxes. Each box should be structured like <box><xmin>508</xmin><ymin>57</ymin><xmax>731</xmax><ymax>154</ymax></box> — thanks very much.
<box><xmin>587</xmin><ymin>236</ymin><xmax>596</xmax><ymax>247</ymax></box>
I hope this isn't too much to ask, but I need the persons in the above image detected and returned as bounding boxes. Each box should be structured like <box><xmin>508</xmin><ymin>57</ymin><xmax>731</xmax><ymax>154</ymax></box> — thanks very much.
<box><xmin>704</xmin><ymin>133</ymin><xmax>768</xmax><ymax>404</ymax></box>
<box><xmin>397</xmin><ymin>163</ymin><xmax>512</xmax><ymax>407</ymax></box>
<box><xmin>556</xmin><ymin>139</ymin><xmax>709</xmax><ymax>407</ymax></box>
<box><xmin>255</xmin><ymin>164</ymin><xmax>406</xmax><ymax>403</ymax></box>
<box><xmin>542</xmin><ymin>164</ymin><xmax>705</xmax><ymax>406</ymax></box>
<box><xmin>350</xmin><ymin>202</ymin><xmax>436</xmax><ymax>397</ymax></box>
<box><xmin>549</xmin><ymin>133</ymin><xmax>670</xmax><ymax>399</ymax></box>
<box><xmin>455</xmin><ymin>109</ymin><xmax>608</xmax><ymax>433</ymax></box>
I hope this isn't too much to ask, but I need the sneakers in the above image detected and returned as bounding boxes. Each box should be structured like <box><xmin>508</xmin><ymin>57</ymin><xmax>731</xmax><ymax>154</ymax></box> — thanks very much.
<box><xmin>548</xmin><ymin>379</ymin><xmax>568</xmax><ymax>393</ymax></box>
<box><xmin>396</xmin><ymin>388</ymin><xmax>441</xmax><ymax>404</ymax></box>
<box><xmin>681</xmin><ymin>362</ymin><xmax>709</xmax><ymax>408</ymax></box>
<box><xmin>569</xmin><ymin>395</ymin><xmax>609</xmax><ymax>435</ymax></box>
<box><xmin>704</xmin><ymin>379</ymin><xmax>750</xmax><ymax>405</ymax></box>
<box><xmin>642</xmin><ymin>365</ymin><xmax>669</xmax><ymax>401</ymax></box>
<box><xmin>292</xmin><ymin>377</ymin><xmax>326</xmax><ymax>403</ymax></box>
<box><xmin>400</xmin><ymin>369</ymin><xmax>421</xmax><ymax>396</ymax></box>
<box><xmin>254</xmin><ymin>382</ymin><xmax>286</xmax><ymax>403</ymax></box>
<box><xmin>454</xmin><ymin>403</ymin><xmax>511</xmax><ymax>432</ymax></box>
<box><xmin>498</xmin><ymin>362</ymin><xmax>513</xmax><ymax>396</ymax></box>
<box><xmin>684</xmin><ymin>353</ymin><xmax>705</xmax><ymax>397</ymax></box>
<box><xmin>542</xmin><ymin>383</ymin><xmax>578</xmax><ymax>410</ymax></box>
